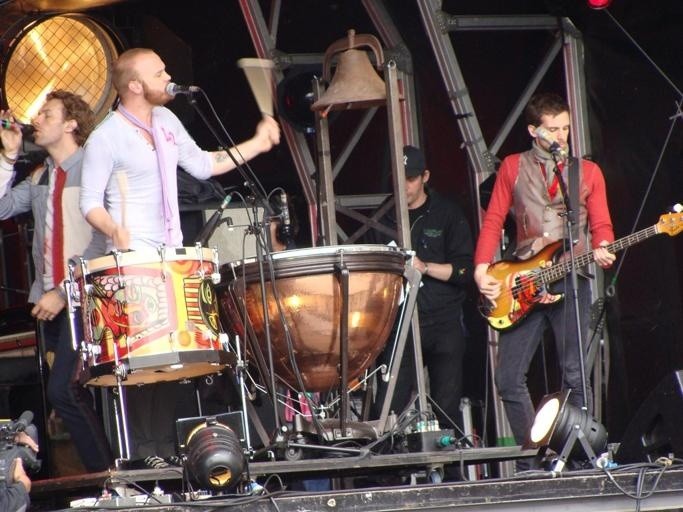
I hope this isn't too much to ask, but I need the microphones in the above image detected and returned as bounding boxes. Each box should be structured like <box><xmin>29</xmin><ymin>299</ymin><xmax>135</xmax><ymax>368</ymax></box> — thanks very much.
<box><xmin>167</xmin><ymin>83</ymin><xmax>198</xmax><ymax>95</ymax></box>
<box><xmin>280</xmin><ymin>194</ymin><xmax>294</xmax><ymax>242</ymax></box>
<box><xmin>536</xmin><ymin>127</ymin><xmax>563</xmax><ymax>156</ymax></box>
<box><xmin>194</xmin><ymin>197</ymin><xmax>231</xmax><ymax>245</ymax></box>
<box><xmin>1</xmin><ymin>117</ymin><xmax>34</xmax><ymax>136</ymax></box>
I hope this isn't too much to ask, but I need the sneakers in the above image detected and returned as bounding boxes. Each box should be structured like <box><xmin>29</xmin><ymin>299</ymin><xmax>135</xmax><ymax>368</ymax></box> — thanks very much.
<box><xmin>164</xmin><ymin>454</ymin><xmax>186</xmax><ymax>467</ymax></box>
<box><xmin>142</xmin><ymin>454</ymin><xmax>172</xmax><ymax>470</ymax></box>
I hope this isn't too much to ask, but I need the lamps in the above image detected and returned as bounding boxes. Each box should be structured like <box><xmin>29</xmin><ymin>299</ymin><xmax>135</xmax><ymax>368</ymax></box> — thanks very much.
<box><xmin>173</xmin><ymin>412</ymin><xmax>255</xmax><ymax>498</ymax></box>
<box><xmin>525</xmin><ymin>389</ymin><xmax>612</xmax><ymax>468</ymax></box>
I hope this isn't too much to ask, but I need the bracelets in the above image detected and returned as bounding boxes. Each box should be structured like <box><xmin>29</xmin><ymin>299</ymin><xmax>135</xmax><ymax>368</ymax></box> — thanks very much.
<box><xmin>0</xmin><ymin>148</ymin><xmax>18</xmax><ymax>166</ymax></box>
<box><xmin>422</xmin><ymin>260</ymin><xmax>429</xmax><ymax>277</ymax></box>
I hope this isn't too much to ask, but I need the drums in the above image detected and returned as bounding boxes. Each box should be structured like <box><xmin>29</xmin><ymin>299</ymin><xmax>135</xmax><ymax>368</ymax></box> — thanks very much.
<box><xmin>214</xmin><ymin>244</ymin><xmax>408</xmax><ymax>392</ymax></box>
<box><xmin>62</xmin><ymin>243</ymin><xmax>232</xmax><ymax>387</ymax></box>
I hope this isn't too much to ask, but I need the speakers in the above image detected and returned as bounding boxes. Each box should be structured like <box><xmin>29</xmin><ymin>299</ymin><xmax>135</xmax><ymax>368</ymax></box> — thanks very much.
<box><xmin>617</xmin><ymin>368</ymin><xmax>682</xmax><ymax>468</ymax></box>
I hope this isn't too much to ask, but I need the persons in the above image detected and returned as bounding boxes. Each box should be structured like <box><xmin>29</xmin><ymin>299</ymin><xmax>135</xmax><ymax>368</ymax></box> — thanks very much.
<box><xmin>352</xmin><ymin>145</ymin><xmax>476</xmax><ymax>488</ymax></box>
<box><xmin>0</xmin><ymin>424</ymin><xmax>39</xmax><ymax>511</ymax></box>
<box><xmin>76</xmin><ymin>44</ymin><xmax>282</xmax><ymax>471</ymax></box>
<box><xmin>0</xmin><ymin>88</ymin><xmax>116</xmax><ymax>475</ymax></box>
<box><xmin>470</xmin><ymin>91</ymin><xmax>618</xmax><ymax>476</ymax></box>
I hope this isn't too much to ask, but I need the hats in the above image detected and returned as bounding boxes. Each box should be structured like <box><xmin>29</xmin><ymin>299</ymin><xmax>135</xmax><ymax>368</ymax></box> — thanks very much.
<box><xmin>403</xmin><ymin>145</ymin><xmax>427</xmax><ymax>179</ymax></box>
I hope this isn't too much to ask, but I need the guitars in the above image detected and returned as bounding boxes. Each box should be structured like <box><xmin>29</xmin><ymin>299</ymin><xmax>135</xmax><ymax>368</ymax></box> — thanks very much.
<box><xmin>479</xmin><ymin>212</ymin><xmax>683</xmax><ymax>331</ymax></box>
